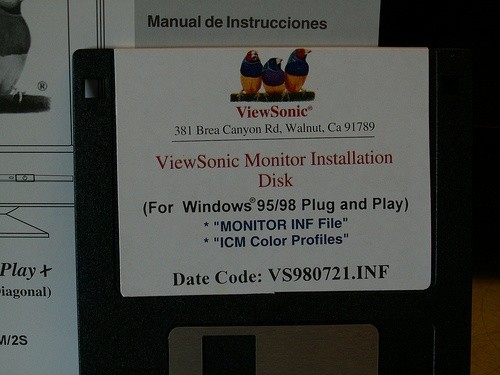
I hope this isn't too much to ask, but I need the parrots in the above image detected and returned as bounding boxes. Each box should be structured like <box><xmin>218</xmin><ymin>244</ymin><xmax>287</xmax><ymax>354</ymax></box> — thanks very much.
<box><xmin>1</xmin><ymin>0</ymin><xmax>32</xmax><ymax>102</ymax></box>
<box><xmin>285</xmin><ymin>48</ymin><xmax>312</xmax><ymax>98</ymax></box>
<box><xmin>237</xmin><ymin>50</ymin><xmax>262</xmax><ymax>101</ymax></box>
<box><xmin>262</xmin><ymin>58</ymin><xmax>287</xmax><ymax>102</ymax></box>
